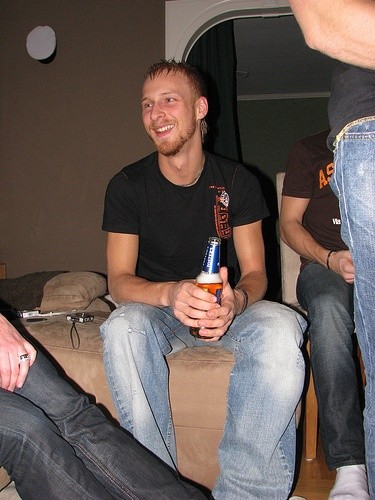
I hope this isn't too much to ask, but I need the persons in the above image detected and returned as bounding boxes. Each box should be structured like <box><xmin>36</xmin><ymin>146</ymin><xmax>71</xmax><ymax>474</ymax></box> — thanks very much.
<box><xmin>98</xmin><ymin>58</ymin><xmax>308</xmax><ymax>500</ymax></box>
<box><xmin>279</xmin><ymin>129</ymin><xmax>371</xmax><ymax>500</ymax></box>
<box><xmin>288</xmin><ymin>0</ymin><xmax>375</xmax><ymax>500</ymax></box>
<box><xmin>0</xmin><ymin>299</ymin><xmax>209</xmax><ymax>500</ymax></box>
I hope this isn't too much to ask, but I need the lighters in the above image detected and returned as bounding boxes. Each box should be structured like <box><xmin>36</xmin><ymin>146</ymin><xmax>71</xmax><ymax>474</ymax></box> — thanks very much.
<box><xmin>27</xmin><ymin>318</ymin><xmax>47</xmax><ymax>322</ymax></box>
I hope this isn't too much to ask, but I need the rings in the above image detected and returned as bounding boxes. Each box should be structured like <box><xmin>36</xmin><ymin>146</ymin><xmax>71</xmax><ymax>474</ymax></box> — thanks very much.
<box><xmin>20</xmin><ymin>354</ymin><xmax>29</xmax><ymax>360</ymax></box>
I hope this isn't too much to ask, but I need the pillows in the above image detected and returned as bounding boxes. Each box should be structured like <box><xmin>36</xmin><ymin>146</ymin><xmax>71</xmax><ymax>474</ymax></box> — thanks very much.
<box><xmin>32</xmin><ymin>267</ymin><xmax>108</xmax><ymax>313</ymax></box>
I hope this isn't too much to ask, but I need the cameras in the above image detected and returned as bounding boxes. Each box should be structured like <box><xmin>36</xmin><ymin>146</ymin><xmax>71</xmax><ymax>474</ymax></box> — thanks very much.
<box><xmin>65</xmin><ymin>313</ymin><xmax>94</xmax><ymax>323</ymax></box>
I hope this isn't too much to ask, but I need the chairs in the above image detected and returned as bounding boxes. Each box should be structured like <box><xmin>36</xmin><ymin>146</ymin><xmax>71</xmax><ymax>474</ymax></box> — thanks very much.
<box><xmin>276</xmin><ymin>170</ymin><xmax>366</xmax><ymax>462</ymax></box>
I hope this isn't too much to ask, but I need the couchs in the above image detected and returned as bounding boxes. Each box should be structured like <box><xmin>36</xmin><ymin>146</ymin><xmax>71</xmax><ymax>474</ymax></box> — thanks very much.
<box><xmin>0</xmin><ymin>263</ymin><xmax>305</xmax><ymax>490</ymax></box>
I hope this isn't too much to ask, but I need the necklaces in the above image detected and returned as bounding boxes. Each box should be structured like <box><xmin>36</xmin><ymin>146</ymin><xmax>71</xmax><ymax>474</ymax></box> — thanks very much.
<box><xmin>181</xmin><ymin>172</ymin><xmax>201</xmax><ymax>186</ymax></box>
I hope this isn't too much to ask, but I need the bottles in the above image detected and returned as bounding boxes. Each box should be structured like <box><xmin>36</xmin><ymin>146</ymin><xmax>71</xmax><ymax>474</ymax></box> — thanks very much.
<box><xmin>190</xmin><ymin>237</ymin><xmax>224</xmax><ymax>340</ymax></box>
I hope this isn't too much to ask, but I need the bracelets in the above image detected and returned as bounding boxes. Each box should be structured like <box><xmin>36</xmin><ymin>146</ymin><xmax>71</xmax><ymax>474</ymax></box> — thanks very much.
<box><xmin>235</xmin><ymin>287</ymin><xmax>248</xmax><ymax>315</ymax></box>
<box><xmin>327</xmin><ymin>251</ymin><xmax>332</xmax><ymax>270</ymax></box>
